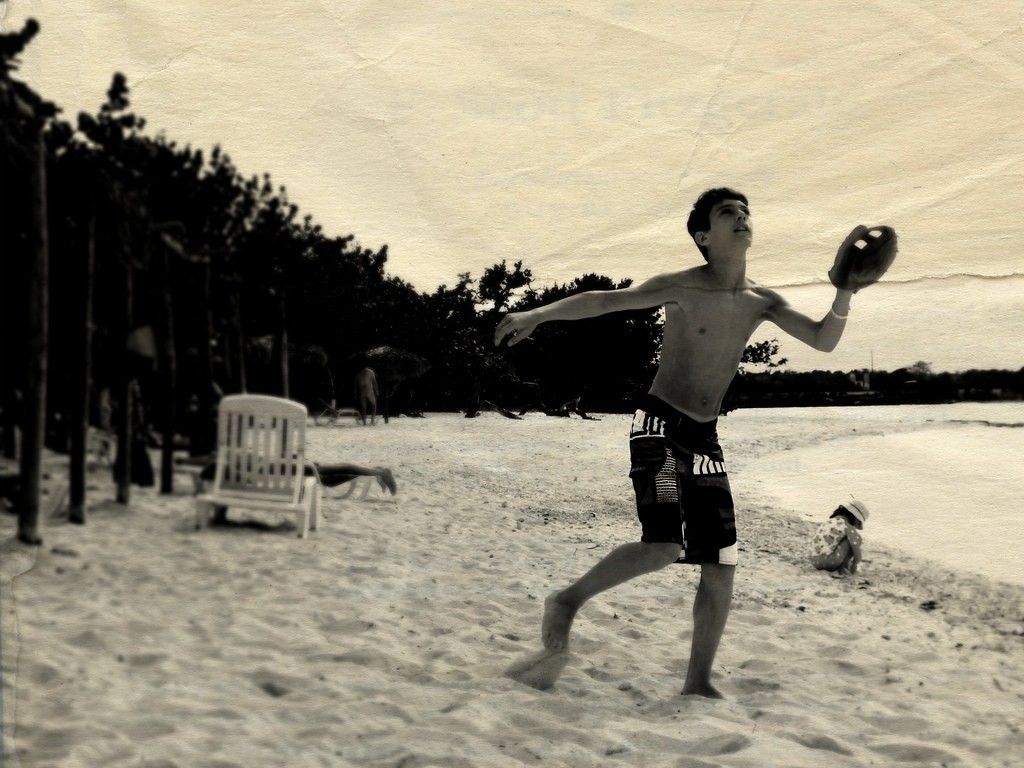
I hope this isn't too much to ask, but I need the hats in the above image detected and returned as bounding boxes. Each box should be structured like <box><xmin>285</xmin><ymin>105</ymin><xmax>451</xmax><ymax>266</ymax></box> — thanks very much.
<box><xmin>840</xmin><ymin>499</ymin><xmax>868</xmax><ymax>530</ymax></box>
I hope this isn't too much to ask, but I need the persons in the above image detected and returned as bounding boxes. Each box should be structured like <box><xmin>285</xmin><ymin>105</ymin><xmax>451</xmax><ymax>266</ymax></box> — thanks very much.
<box><xmin>353</xmin><ymin>363</ymin><xmax>379</xmax><ymax>426</ymax></box>
<box><xmin>810</xmin><ymin>498</ymin><xmax>870</xmax><ymax>578</ymax></box>
<box><xmin>494</xmin><ymin>185</ymin><xmax>859</xmax><ymax>704</ymax></box>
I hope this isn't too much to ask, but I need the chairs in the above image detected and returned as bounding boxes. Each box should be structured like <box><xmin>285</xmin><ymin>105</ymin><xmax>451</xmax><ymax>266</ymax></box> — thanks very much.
<box><xmin>173</xmin><ymin>382</ymin><xmax>397</xmax><ymax>538</ymax></box>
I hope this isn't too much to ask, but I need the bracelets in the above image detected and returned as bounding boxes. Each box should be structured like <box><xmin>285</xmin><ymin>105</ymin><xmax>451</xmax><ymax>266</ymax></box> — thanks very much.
<box><xmin>829</xmin><ymin>304</ymin><xmax>850</xmax><ymax>320</ymax></box>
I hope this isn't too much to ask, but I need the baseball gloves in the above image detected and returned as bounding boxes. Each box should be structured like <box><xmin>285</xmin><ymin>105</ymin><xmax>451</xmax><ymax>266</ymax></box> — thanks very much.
<box><xmin>828</xmin><ymin>223</ymin><xmax>898</xmax><ymax>294</ymax></box>
